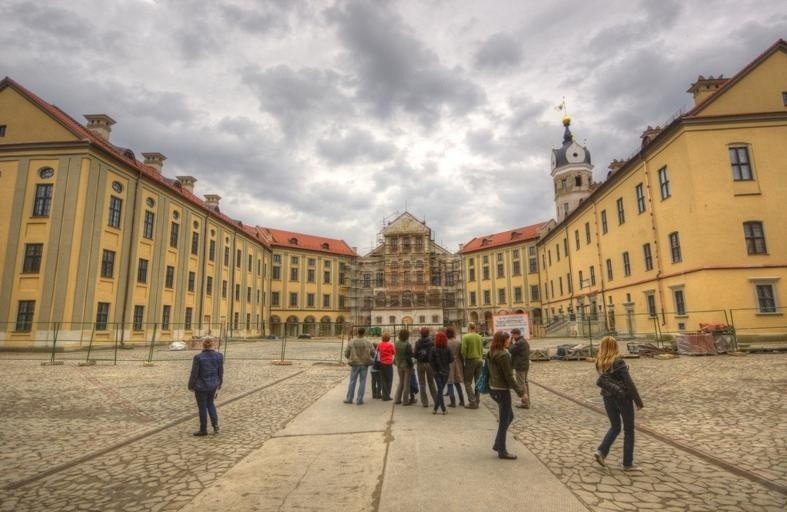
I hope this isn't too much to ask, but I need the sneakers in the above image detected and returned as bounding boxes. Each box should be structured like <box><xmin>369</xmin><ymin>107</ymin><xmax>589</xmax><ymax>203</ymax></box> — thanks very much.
<box><xmin>623</xmin><ymin>464</ymin><xmax>643</xmax><ymax>471</ymax></box>
<box><xmin>193</xmin><ymin>431</ymin><xmax>208</xmax><ymax>436</ymax></box>
<box><xmin>493</xmin><ymin>445</ymin><xmax>500</xmax><ymax>452</ymax></box>
<box><xmin>498</xmin><ymin>453</ymin><xmax>517</xmax><ymax>459</ymax></box>
<box><xmin>214</xmin><ymin>425</ymin><xmax>219</xmax><ymax>433</ymax></box>
<box><xmin>594</xmin><ymin>452</ymin><xmax>605</xmax><ymax>466</ymax></box>
<box><xmin>515</xmin><ymin>400</ymin><xmax>529</xmax><ymax>409</ymax></box>
<box><xmin>343</xmin><ymin>392</ymin><xmax>479</xmax><ymax>415</ymax></box>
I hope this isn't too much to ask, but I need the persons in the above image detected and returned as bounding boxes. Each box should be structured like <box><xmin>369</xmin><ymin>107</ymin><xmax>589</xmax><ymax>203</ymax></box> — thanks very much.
<box><xmin>592</xmin><ymin>335</ymin><xmax>645</xmax><ymax>472</ymax></box>
<box><xmin>444</xmin><ymin>327</ymin><xmax>466</xmax><ymax>408</ymax></box>
<box><xmin>407</xmin><ymin>356</ymin><xmax>418</xmax><ymax>405</ymax></box>
<box><xmin>394</xmin><ymin>328</ymin><xmax>412</xmax><ymax>408</ymax></box>
<box><xmin>430</xmin><ymin>331</ymin><xmax>453</xmax><ymax>415</ymax></box>
<box><xmin>413</xmin><ymin>326</ymin><xmax>440</xmax><ymax>406</ymax></box>
<box><xmin>370</xmin><ymin>342</ymin><xmax>381</xmax><ymax>399</ymax></box>
<box><xmin>343</xmin><ymin>327</ymin><xmax>371</xmax><ymax>405</ymax></box>
<box><xmin>507</xmin><ymin>328</ymin><xmax>531</xmax><ymax>409</ymax></box>
<box><xmin>375</xmin><ymin>331</ymin><xmax>396</xmax><ymax>401</ymax></box>
<box><xmin>477</xmin><ymin>331</ymin><xmax>531</xmax><ymax>461</ymax></box>
<box><xmin>186</xmin><ymin>337</ymin><xmax>225</xmax><ymax>437</ymax></box>
<box><xmin>459</xmin><ymin>319</ymin><xmax>481</xmax><ymax>409</ymax></box>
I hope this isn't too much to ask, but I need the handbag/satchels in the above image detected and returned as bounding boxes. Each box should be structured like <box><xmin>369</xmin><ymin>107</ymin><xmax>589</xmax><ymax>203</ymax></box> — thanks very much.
<box><xmin>597</xmin><ymin>365</ymin><xmax>628</xmax><ymax>400</ymax></box>
<box><xmin>475</xmin><ymin>360</ymin><xmax>490</xmax><ymax>393</ymax></box>
<box><xmin>373</xmin><ymin>361</ymin><xmax>380</xmax><ymax>370</ymax></box>
